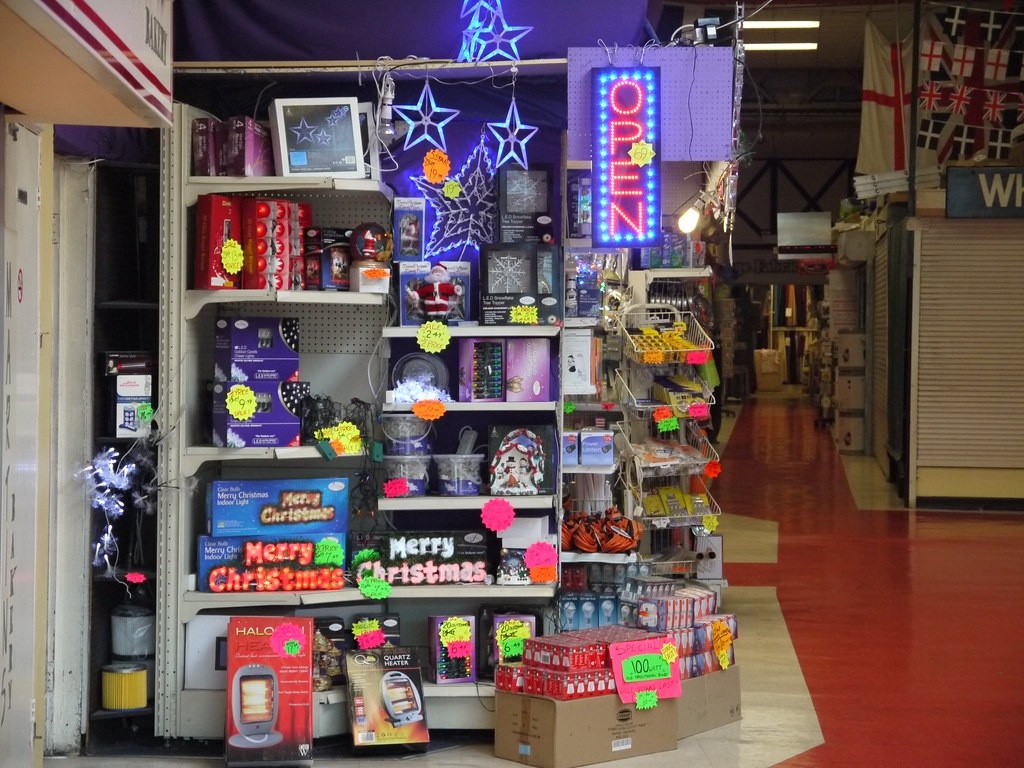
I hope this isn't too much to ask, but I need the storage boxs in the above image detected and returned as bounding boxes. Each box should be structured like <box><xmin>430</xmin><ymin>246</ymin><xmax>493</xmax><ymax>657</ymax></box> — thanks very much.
<box><xmin>359</xmin><ymin>102</ymin><xmax>382</xmax><ymax>181</ymax></box>
<box><xmin>493</xmin><ymin>689</ymin><xmax>677</xmax><ymax>768</ymax></box>
<box><xmin>496</xmin><ymin>548</ymin><xmax>535</xmax><ymax>585</ymax></box>
<box><xmin>501</xmin><ymin>533</ymin><xmax>557</xmax><ymax>548</ymax></box>
<box><xmin>458</xmin><ymin>161</ymin><xmax>739</xmax><ymax>701</ymax></box>
<box><xmin>224</xmin><ymin>616</ymin><xmax>315</xmax><ymax>768</ymax></box>
<box><xmin>190</xmin><ymin>115</ymin><xmax>352</xmax><ymax>292</ymax></box>
<box><xmin>196</xmin><ymin>534</ymin><xmax>346</xmax><ymax>593</ymax></box>
<box><xmin>342</xmin><ymin>646</ymin><xmax>430</xmax><ymax>758</ymax></box>
<box><xmin>100</xmin><ymin>349</ymin><xmax>301</xmax><ymax>448</ymax></box>
<box><xmin>268</xmin><ymin>96</ymin><xmax>366</xmax><ymax>179</ymax></box>
<box><xmin>314</xmin><ymin>613</ymin><xmax>401</xmax><ymax>687</ymax></box>
<box><xmin>427</xmin><ymin>615</ymin><xmax>476</xmax><ymax>685</ymax></box>
<box><xmin>348</xmin><ymin>525</ymin><xmax>488</xmax><ymax>588</ymax></box>
<box><xmin>392</xmin><ymin>197</ymin><xmax>426</xmax><ymax>263</ymax></box>
<box><xmin>398</xmin><ymin>260</ymin><xmax>471</xmax><ymax>328</ymax></box>
<box><xmin>203</xmin><ymin>476</ymin><xmax>350</xmax><ymax>538</ymax></box>
<box><xmin>497</xmin><ymin>514</ymin><xmax>549</xmax><ymax>539</ymax></box>
<box><xmin>212</xmin><ymin>316</ymin><xmax>301</xmax><ymax>383</ymax></box>
<box><xmin>480</xmin><ymin>612</ymin><xmax>535</xmax><ymax>684</ymax></box>
<box><xmin>677</xmin><ymin>664</ymin><xmax>743</xmax><ymax>740</ymax></box>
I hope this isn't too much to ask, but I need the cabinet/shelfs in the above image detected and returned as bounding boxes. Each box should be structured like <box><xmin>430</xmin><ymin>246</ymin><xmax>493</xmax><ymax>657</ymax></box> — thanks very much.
<box><xmin>565</xmin><ymin>317</ymin><xmax>638</xmax><ymax>564</ymax></box>
<box><xmin>625</xmin><ymin>263</ymin><xmax>729</xmax><ymax>613</ymax></box>
<box><xmin>89</xmin><ymin>299</ymin><xmax>158</xmax><ymax>722</ymax></box>
<box><xmin>153</xmin><ymin>103</ymin><xmax>560</xmax><ymax>758</ymax></box>
